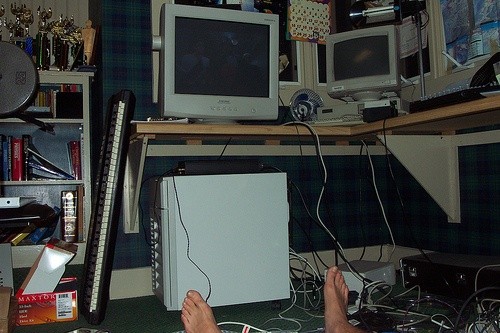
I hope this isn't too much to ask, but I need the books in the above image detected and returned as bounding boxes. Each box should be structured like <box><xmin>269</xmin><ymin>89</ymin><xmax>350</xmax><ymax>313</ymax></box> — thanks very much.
<box><xmin>0</xmin><ymin>133</ymin><xmax>84</xmax><ymax>181</ymax></box>
<box><xmin>32</xmin><ymin>84</ymin><xmax>81</xmax><ymax>119</ymax></box>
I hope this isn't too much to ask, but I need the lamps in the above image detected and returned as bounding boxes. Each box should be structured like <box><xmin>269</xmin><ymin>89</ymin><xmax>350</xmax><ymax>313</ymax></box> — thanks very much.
<box><xmin>349</xmin><ymin>0</ymin><xmax>426</xmax><ymax>97</ymax></box>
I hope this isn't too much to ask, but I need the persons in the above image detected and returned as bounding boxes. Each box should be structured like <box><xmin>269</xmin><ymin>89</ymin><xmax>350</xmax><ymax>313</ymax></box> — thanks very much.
<box><xmin>181</xmin><ymin>265</ymin><xmax>365</xmax><ymax>333</ymax></box>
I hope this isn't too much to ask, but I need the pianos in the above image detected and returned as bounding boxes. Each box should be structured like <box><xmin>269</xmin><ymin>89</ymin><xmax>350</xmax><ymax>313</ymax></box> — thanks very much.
<box><xmin>77</xmin><ymin>89</ymin><xmax>137</xmax><ymax>326</ymax></box>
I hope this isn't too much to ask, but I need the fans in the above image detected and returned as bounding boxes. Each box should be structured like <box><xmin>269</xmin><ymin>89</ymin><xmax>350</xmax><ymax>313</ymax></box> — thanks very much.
<box><xmin>290</xmin><ymin>89</ymin><xmax>323</xmax><ymax>122</ymax></box>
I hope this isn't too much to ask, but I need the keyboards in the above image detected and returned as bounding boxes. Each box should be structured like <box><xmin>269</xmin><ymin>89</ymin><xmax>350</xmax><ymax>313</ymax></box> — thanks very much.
<box><xmin>305</xmin><ymin>116</ymin><xmax>366</xmax><ymax>126</ymax></box>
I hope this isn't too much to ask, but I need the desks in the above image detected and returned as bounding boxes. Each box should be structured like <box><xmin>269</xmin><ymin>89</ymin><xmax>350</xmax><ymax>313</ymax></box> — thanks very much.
<box><xmin>123</xmin><ymin>94</ymin><xmax>500</xmax><ymax>143</ymax></box>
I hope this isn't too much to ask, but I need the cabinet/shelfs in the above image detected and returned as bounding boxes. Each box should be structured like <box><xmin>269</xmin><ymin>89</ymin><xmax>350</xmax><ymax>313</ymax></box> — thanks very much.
<box><xmin>0</xmin><ymin>71</ymin><xmax>91</xmax><ymax>268</ymax></box>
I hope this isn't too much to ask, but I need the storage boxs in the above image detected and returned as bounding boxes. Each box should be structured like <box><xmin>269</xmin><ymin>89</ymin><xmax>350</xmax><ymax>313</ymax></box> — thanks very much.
<box><xmin>15</xmin><ymin>237</ymin><xmax>78</xmax><ymax>325</ymax></box>
<box><xmin>0</xmin><ymin>287</ymin><xmax>17</xmax><ymax>333</ymax></box>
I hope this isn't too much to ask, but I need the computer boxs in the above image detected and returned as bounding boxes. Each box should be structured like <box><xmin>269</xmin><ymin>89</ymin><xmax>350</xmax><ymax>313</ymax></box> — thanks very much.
<box><xmin>149</xmin><ymin>172</ymin><xmax>292</xmax><ymax>312</ymax></box>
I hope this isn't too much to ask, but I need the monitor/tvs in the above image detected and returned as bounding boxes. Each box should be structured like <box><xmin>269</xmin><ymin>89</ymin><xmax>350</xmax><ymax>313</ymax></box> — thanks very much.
<box><xmin>158</xmin><ymin>2</ymin><xmax>279</xmax><ymax>125</ymax></box>
<box><xmin>325</xmin><ymin>24</ymin><xmax>402</xmax><ymax>102</ymax></box>
